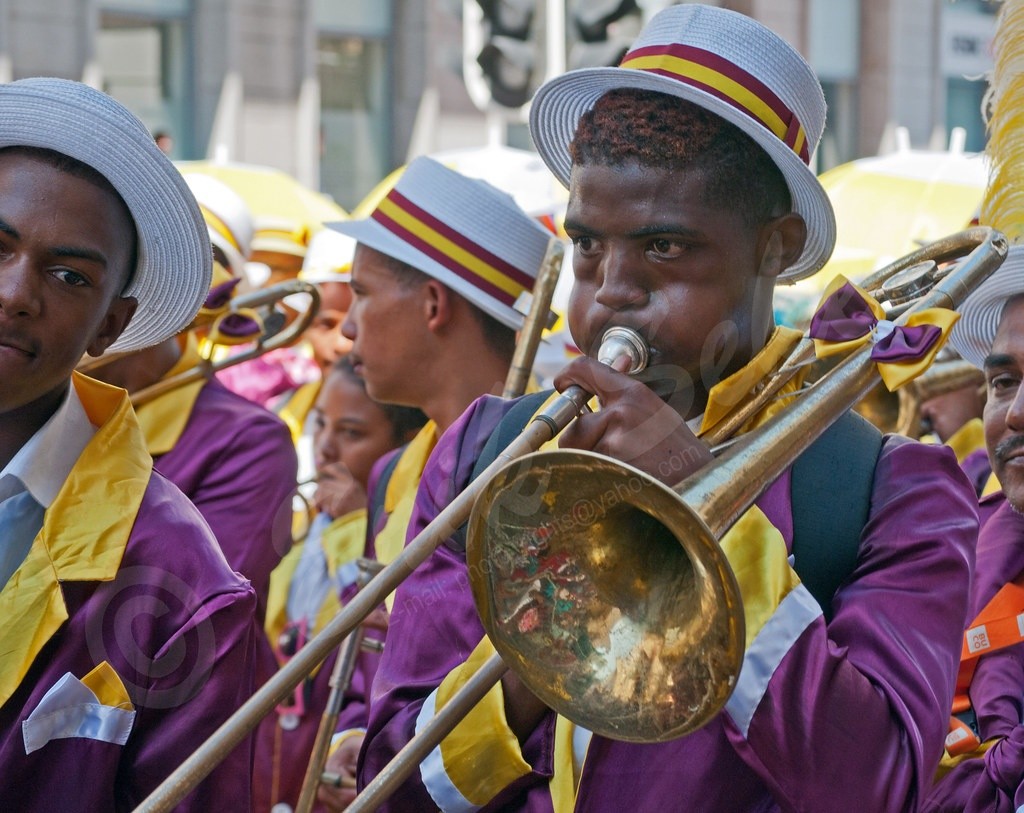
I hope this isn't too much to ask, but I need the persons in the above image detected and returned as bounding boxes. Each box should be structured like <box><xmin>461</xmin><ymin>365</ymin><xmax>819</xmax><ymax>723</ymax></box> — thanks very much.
<box><xmin>84</xmin><ymin>134</ymin><xmax>1011</xmax><ymax>576</ymax></box>
<box><xmin>0</xmin><ymin>73</ymin><xmax>258</xmax><ymax>813</ymax></box>
<box><xmin>354</xmin><ymin>3</ymin><xmax>981</xmax><ymax>813</ymax></box>
<box><xmin>252</xmin><ymin>353</ymin><xmax>431</xmax><ymax>813</ymax></box>
<box><xmin>268</xmin><ymin>228</ymin><xmax>354</xmax><ymax>638</ymax></box>
<box><xmin>915</xmin><ymin>246</ymin><xmax>1024</xmax><ymax>813</ymax></box>
<box><xmin>314</xmin><ymin>158</ymin><xmax>561</xmax><ymax>813</ymax></box>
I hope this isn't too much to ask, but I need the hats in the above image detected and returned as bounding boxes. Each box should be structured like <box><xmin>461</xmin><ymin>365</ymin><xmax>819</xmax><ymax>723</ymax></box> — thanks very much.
<box><xmin>299</xmin><ymin>236</ymin><xmax>353</xmax><ymax>280</ymax></box>
<box><xmin>181</xmin><ymin>173</ymin><xmax>253</xmax><ymax>271</ymax></box>
<box><xmin>530</xmin><ymin>5</ymin><xmax>837</xmax><ymax>285</ymax></box>
<box><xmin>0</xmin><ymin>78</ymin><xmax>211</xmax><ymax>353</ymax></box>
<box><xmin>948</xmin><ymin>243</ymin><xmax>1023</xmax><ymax>371</ymax></box>
<box><xmin>320</xmin><ymin>156</ymin><xmax>563</xmax><ymax>347</ymax></box>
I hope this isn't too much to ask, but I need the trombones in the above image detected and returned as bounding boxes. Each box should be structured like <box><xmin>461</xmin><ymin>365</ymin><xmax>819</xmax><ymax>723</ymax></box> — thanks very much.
<box><xmin>75</xmin><ymin>279</ymin><xmax>320</xmax><ymax>409</ymax></box>
<box><xmin>293</xmin><ymin>237</ymin><xmax>566</xmax><ymax>813</ymax></box>
<box><xmin>129</xmin><ymin>225</ymin><xmax>1011</xmax><ymax>813</ymax></box>
<box><xmin>851</xmin><ymin>361</ymin><xmax>986</xmax><ymax>443</ymax></box>
